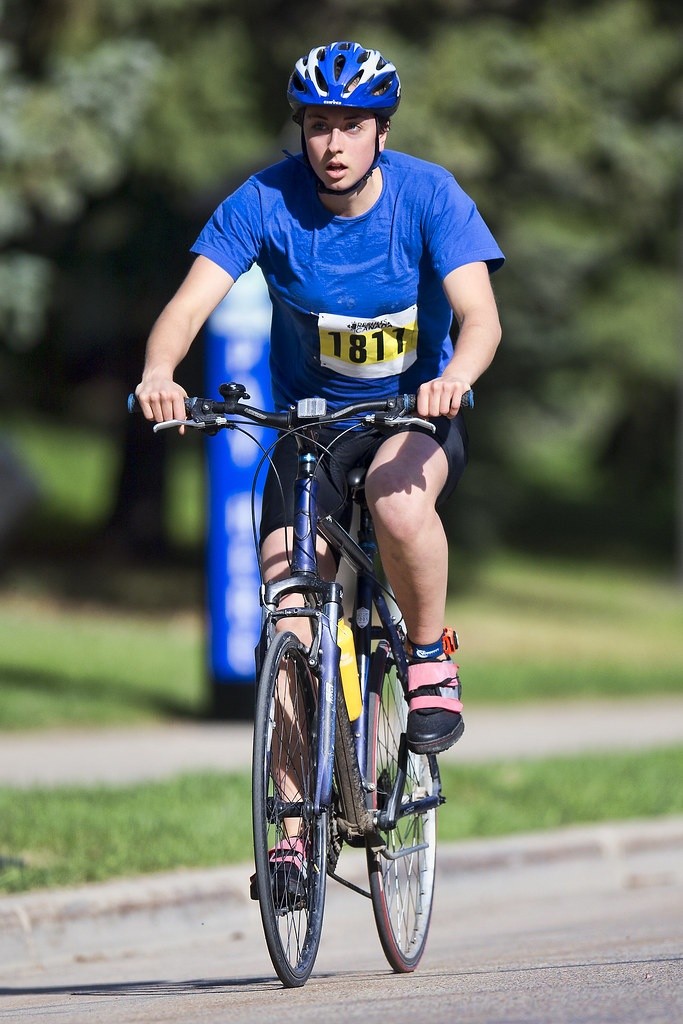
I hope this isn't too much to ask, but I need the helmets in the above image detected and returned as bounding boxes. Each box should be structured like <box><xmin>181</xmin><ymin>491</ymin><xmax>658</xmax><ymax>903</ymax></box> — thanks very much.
<box><xmin>286</xmin><ymin>41</ymin><xmax>402</xmax><ymax>114</ymax></box>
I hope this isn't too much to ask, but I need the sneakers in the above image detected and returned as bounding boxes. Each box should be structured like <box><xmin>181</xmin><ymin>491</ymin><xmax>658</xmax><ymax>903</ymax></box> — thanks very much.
<box><xmin>250</xmin><ymin>836</ymin><xmax>307</xmax><ymax>902</ymax></box>
<box><xmin>405</xmin><ymin>658</ymin><xmax>466</xmax><ymax>754</ymax></box>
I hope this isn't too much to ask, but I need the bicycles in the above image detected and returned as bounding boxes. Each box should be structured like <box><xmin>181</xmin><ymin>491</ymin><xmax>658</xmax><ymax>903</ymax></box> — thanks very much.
<box><xmin>127</xmin><ymin>379</ymin><xmax>474</xmax><ymax>988</ymax></box>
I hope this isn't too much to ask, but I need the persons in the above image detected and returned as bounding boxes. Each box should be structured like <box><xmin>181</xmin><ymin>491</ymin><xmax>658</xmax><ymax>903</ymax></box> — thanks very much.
<box><xmin>136</xmin><ymin>39</ymin><xmax>509</xmax><ymax>899</ymax></box>
